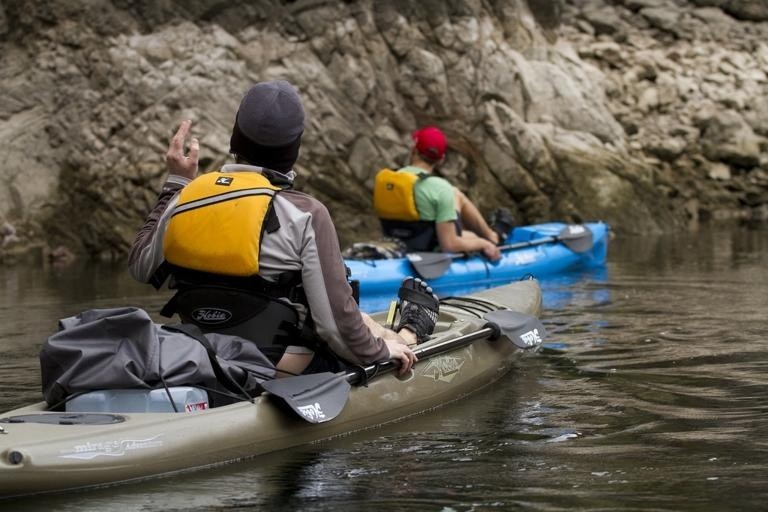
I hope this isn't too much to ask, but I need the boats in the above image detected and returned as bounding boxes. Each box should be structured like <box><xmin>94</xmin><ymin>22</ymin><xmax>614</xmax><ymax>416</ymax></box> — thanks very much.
<box><xmin>0</xmin><ymin>277</ymin><xmax>543</xmax><ymax>501</ymax></box>
<box><xmin>341</xmin><ymin>221</ymin><xmax>608</xmax><ymax>297</ymax></box>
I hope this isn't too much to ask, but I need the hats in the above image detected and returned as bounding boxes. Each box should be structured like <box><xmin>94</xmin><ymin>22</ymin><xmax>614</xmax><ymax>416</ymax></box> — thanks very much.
<box><xmin>410</xmin><ymin>125</ymin><xmax>449</xmax><ymax>162</ymax></box>
<box><xmin>224</xmin><ymin>79</ymin><xmax>305</xmax><ymax>175</ymax></box>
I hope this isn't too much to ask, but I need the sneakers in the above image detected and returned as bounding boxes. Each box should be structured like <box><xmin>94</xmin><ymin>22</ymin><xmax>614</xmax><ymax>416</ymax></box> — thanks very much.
<box><xmin>487</xmin><ymin>206</ymin><xmax>514</xmax><ymax>243</ymax></box>
<box><xmin>395</xmin><ymin>274</ymin><xmax>441</xmax><ymax>346</ymax></box>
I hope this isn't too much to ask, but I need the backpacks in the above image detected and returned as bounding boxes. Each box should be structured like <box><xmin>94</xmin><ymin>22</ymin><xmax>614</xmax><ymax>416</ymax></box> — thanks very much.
<box><xmin>36</xmin><ymin>306</ymin><xmax>277</xmax><ymax>412</ymax></box>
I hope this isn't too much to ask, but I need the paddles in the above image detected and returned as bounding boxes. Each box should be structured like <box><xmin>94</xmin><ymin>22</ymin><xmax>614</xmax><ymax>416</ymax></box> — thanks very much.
<box><xmin>406</xmin><ymin>224</ymin><xmax>593</xmax><ymax>280</ymax></box>
<box><xmin>260</xmin><ymin>310</ymin><xmax>547</xmax><ymax>424</ymax></box>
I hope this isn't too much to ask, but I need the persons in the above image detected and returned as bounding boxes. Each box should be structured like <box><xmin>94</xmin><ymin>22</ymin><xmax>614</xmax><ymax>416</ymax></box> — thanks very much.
<box><xmin>371</xmin><ymin>126</ymin><xmax>516</xmax><ymax>263</ymax></box>
<box><xmin>129</xmin><ymin>79</ymin><xmax>440</xmax><ymax>377</ymax></box>
